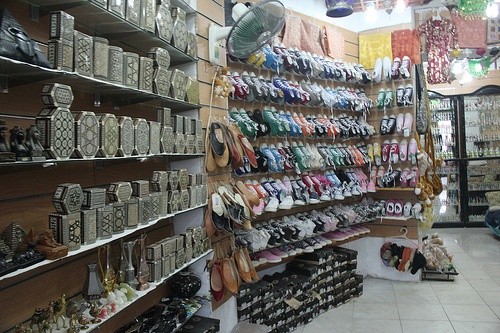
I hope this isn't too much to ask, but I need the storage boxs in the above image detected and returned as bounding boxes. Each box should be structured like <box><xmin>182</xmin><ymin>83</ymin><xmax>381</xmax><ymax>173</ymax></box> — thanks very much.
<box><xmin>181</xmin><ymin>315</ymin><xmax>220</xmax><ymax>333</ymax></box>
<box><xmin>235</xmin><ymin>246</ymin><xmax>364</xmax><ymax>333</ymax></box>
<box><xmin>36</xmin><ymin>0</ymin><xmax>212</xmax><ymax>283</ymax></box>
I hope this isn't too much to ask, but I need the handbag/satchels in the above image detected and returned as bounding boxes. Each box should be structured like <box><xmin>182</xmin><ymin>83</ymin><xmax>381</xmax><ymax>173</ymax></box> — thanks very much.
<box><xmin>414</xmin><ymin>63</ymin><xmax>442</xmax><ymax>207</ymax></box>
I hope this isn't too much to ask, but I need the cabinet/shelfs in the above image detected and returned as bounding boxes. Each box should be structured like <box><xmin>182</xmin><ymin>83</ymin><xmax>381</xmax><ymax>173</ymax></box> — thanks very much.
<box><xmin>428</xmin><ymin>94</ymin><xmax>500</xmax><ymax>228</ymax></box>
<box><xmin>225</xmin><ymin>43</ymin><xmax>424</xmax><ymax>273</ymax></box>
<box><xmin>0</xmin><ymin>0</ymin><xmax>215</xmax><ymax>333</ymax></box>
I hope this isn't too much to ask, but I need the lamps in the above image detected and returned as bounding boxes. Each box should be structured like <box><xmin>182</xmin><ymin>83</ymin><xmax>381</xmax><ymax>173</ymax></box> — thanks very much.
<box><xmin>325</xmin><ymin>0</ymin><xmax>353</xmax><ymax>17</ymax></box>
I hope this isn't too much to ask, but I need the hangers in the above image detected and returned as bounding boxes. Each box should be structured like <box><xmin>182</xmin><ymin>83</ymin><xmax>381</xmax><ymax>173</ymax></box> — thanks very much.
<box><xmin>390</xmin><ymin>227</ymin><xmax>419</xmax><ymax>249</ymax></box>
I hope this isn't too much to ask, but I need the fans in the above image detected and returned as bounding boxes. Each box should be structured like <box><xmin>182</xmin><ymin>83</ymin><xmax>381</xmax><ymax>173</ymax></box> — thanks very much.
<box><xmin>208</xmin><ymin>0</ymin><xmax>285</xmax><ymax>66</ymax></box>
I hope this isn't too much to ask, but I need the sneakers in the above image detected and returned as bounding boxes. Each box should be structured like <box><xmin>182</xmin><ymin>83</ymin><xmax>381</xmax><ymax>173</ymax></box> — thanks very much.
<box><xmin>205</xmin><ymin>42</ymin><xmax>419</xmax><ymax>302</ymax></box>
